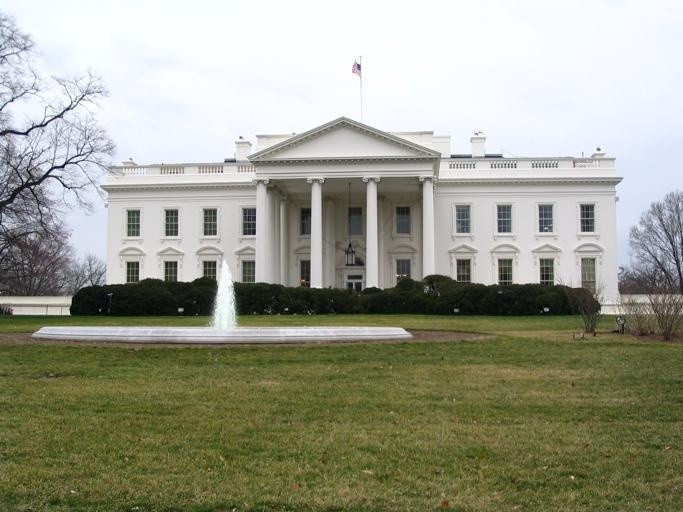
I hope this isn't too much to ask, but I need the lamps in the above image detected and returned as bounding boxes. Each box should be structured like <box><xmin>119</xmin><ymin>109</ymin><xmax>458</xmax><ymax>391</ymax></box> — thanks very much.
<box><xmin>345</xmin><ymin>182</ymin><xmax>355</xmax><ymax>266</ymax></box>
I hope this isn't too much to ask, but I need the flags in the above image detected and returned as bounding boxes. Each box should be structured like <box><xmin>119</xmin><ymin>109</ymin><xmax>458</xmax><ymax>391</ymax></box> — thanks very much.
<box><xmin>352</xmin><ymin>59</ymin><xmax>360</xmax><ymax>76</ymax></box>
